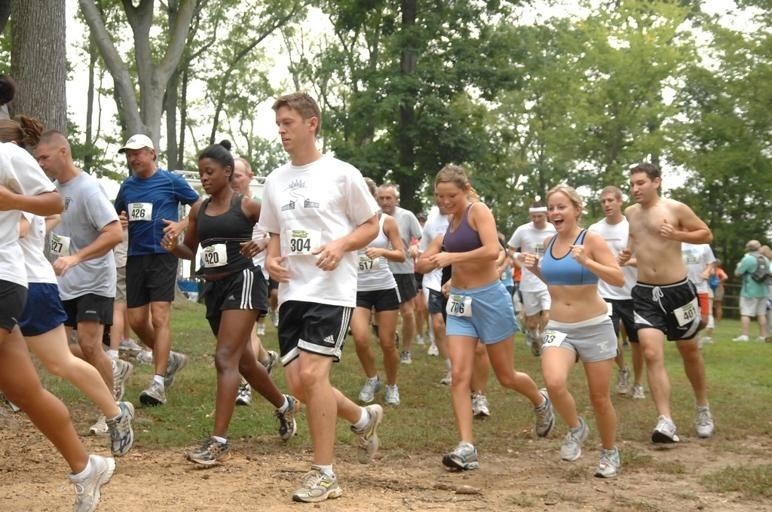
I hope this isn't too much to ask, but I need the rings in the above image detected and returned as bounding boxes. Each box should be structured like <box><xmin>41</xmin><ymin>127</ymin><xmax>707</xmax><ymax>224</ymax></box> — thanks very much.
<box><xmin>331</xmin><ymin>260</ymin><xmax>336</xmax><ymax>266</ymax></box>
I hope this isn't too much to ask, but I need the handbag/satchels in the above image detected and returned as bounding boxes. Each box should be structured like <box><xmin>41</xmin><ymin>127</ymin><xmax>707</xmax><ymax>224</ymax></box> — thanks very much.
<box><xmin>709</xmin><ymin>274</ymin><xmax>720</xmax><ymax>290</ymax></box>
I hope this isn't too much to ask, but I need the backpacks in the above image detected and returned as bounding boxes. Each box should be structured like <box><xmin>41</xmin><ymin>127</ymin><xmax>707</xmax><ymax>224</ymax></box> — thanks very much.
<box><xmin>751</xmin><ymin>255</ymin><xmax>769</xmax><ymax>284</ymax></box>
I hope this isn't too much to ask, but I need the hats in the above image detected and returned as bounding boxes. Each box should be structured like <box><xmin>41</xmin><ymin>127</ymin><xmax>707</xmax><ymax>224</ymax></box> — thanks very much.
<box><xmin>744</xmin><ymin>239</ymin><xmax>761</xmax><ymax>251</ymax></box>
<box><xmin>118</xmin><ymin>134</ymin><xmax>155</xmax><ymax>154</ymax></box>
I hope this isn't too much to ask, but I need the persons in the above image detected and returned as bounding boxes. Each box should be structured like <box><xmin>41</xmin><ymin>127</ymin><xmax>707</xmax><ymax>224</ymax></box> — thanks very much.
<box><xmin>161</xmin><ymin>139</ymin><xmax>300</xmax><ymax>465</ymax></box>
<box><xmin>0</xmin><ymin>74</ymin><xmax>117</xmax><ymax>512</ymax></box>
<box><xmin>32</xmin><ymin>128</ymin><xmax>132</xmax><ymax>438</ymax></box>
<box><xmin>355</xmin><ymin>177</ymin><xmax>772</xmax><ymax>403</ymax></box>
<box><xmin>113</xmin><ymin>132</ymin><xmax>200</xmax><ymax>404</ymax></box>
<box><xmin>221</xmin><ymin>156</ymin><xmax>279</xmax><ymax>406</ymax></box>
<box><xmin>101</xmin><ymin>216</ymin><xmax>156</xmax><ymax>370</ymax></box>
<box><xmin>523</xmin><ymin>181</ymin><xmax>629</xmax><ymax>482</ymax></box>
<box><xmin>415</xmin><ymin>161</ymin><xmax>556</xmax><ymax>474</ymax></box>
<box><xmin>259</xmin><ymin>91</ymin><xmax>386</xmax><ymax>506</ymax></box>
<box><xmin>618</xmin><ymin>161</ymin><xmax>716</xmax><ymax>446</ymax></box>
<box><xmin>0</xmin><ymin>114</ymin><xmax>137</xmax><ymax>456</ymax></box>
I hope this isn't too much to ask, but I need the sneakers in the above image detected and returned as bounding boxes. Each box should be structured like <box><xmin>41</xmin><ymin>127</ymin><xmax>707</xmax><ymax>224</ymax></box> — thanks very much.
<box><xmin>272</xmin><ymin>312</ymin><xmax>279</xmax><ymax>328</ymax></box>
<box><xmin>400</xmin><ymin>351</ymin><xmax>413</xmax><ymax>365</ymax></box>
<box><xmin>183</xmin><ymin>438</ymin><xmax>232</xmax><ymax>465</ymax></box>
<box><xmin>471</xmin><ymin>394</ymin><xmax>491</xmax><ymax>416</ymax></box>
<box><xmin>534</xmin><ymin>387</ymin><xmax>555</xmax><ymax>438</ymax></box>
<box><xmin>394</xmin><ymin>330</ymin><xmax>399</xmax><ymax>349</ymax></box>
<box><xmin>139</xmin><ymin>380</ymin><xmax>167</xmax><ymax>406</ymax></box>
<box><xmin>118</xmin><ymin>338</ymin><xmax>144</xmax><ymax>350</ymax></box>
<box><xmin>427</xmin><ymin>343</ymin><xmax>440</xmax><ymax>357</ymax></box>
<box><xmin>292</xmin><ymin>466</ymin><xmax>344</xmax><ymax>503</ymax></box>
<box><xmin>632</xmin><ymin>384</ymin><xmax>646</xmax><ymax>399</ymax></box>
<box><xmin>652</xmin><ymin>415</ymin><xmax>677</xmax><ymax>442</ymax></box>
<box><xmin>350</xmin><ymin>403</ymin><xmax>384</xmax><ymax>464</ymax></box>
<box><xmin>104</xmin><ymin>401</ymin><xmax>135</xmax><ymax>457</ymax></box>
<box><xmin>164</xmin><ymin>351</ymin><xmax>188</xmax><ymax>393</ymax></box>
<box><xmin>111</xmin><ymin>359</ymin><xmax>133</xmax><ymax>401</ymax></box>
<box><xmin>731</xmin><ymin>335</ymin><xmax>749</xmax><ymax>342</ymax></box>
<box><xmin>88</xmin><ymin>415</ymin><xmax>109</xmax><ymax>435</ymax></box>
<box><xmin>440</xmin><ymin>372</ymin><xmax>451</xmax><ymax>385</ymax></box>
<box><xmin>358</xmin><ymin>374</ymin><xmax>381</xmax><ymax>403</ymax></box>
<box><xmin>385</xmin><ymin>384</ymin><xmax>400</xmax><ymax>406</ymax></box>
<box><xmin>416</xmin><ymin>333</ymin><xmax>425</xmax><ymax>345</ymax></box>
<box><xmin>755</xmin><ymin>336</ymin><xmax>766</xmax><ymax>343</ymax></box>
<box><xmin>67</xmin><ymin>454</ymin><xmax>116</xmax><ymax>512</ymax></box>
<box><xmin>560</xmin><ymin>415</ymin><xmax>590</xmax><ymax>462</ymax></box>
<box><xmin>442</xmin><ymin>444</ymin><xmax>480</xmax><ymax>470</ymax></box>
<box><xmin>257</xmin><ymin>324</ymin><xmax>266</xmax><ymax>335</ymax></box>
<box><xmin>137</xmin><ymin>351</ymin><xmax>153</xmax><ymax>366</ymax></box>
<box><xmin>615</xmin><ymin>367</ymin><xmax>631</xmax><ymax>394</ymax></box>
<box><xmin>262</xmin><ymin>350</ymin><xmax>279</xmax><ymax>375</ymax></box>
<box><xmin>531</xmin><ymin>342</ymin><xmax>542</xmax><ymax>356</ymax></box>
<box><xmin>694</xmin><ymin>400</ymin><xmax>714</xmax><ymax>438</ymax></box>
<box><xmin>235</xmin><ymin>384</ymin><xmax>252</xmax><ymax>406</ymax></box>
<box><xmin>274</xmin><ymin>394</ymin><xmax>301</xmax><ymax>442</ymax></box>
<box><xmin>593</xmin><ymin>446</ymin><xmax>621</xmax><ymax>478</ymax></box>
